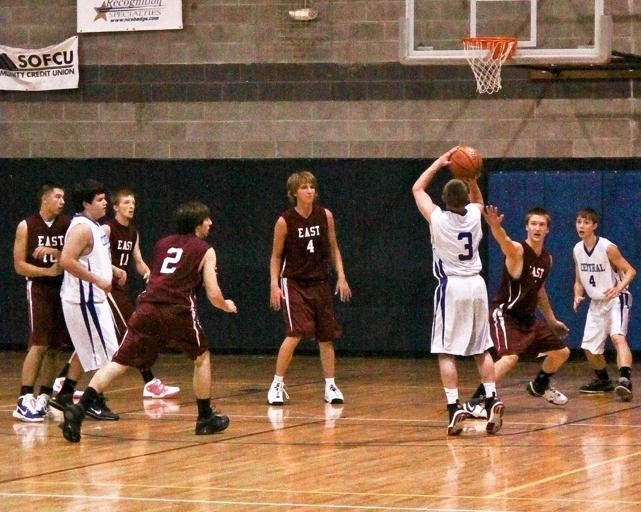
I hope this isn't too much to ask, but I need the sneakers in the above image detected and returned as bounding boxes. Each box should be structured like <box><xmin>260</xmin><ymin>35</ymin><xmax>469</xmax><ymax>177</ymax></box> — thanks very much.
<box><xmin>485</xmin><ymin>395</ymin><xmax>507</xmax><ymax>437</ymax></box>
<box><xmin>141</xmin><ymin>376</ymin><xmax>181</xmax><ymax>400</ymax></box>
<box><xmin>524</xmin><ymin>380</ymin><xmax>570</xmax><ymax>406</ymax></box>
<box><xmin>61</xmin><ymin>403</ymin><xmax>83</xmax><ymax>442</ymax></box>
<box><xmin>11</xmin><ymin>390</ymin><xmax>51</xmax><ymax>423</ymax></box>
<box><xmin>264</xmin><ymin>382</ymin><xmax>285</xmax><ymax>406</ymax></box>
<box><xmin>462</xmin><ymin>395</ymin><xmax>489</xmax><ymax>419</ymax></box>
<box><xmin>614</xmin><ymin>377</ymin><xmax>634</xmax><ymax>404</ymax></box>
<box><xmin>50</xmin><ymin>375</ymin><xmax>85</xmax><ymax>401</ymax></box>
<box><xmin>577</xmin><ymin>377</ymin><xmax>616</xmax><ymax>394</ymax></box>
<box><xmin>443</xmin><ymin>402</ymin><xmax>468</xmax><ymax>436</ymax></box>
<box><xmin>47</xmin><ymin>392</ymin><xmax>79</xmax><ymax>411</ymax></box>
<box><xmin>194</xmin><ymin>415</ymin><xmax>230</xmax><ymax>435</ymax></box>
<box><xmin>321</xmin><ymin>384</ymin><xmax>345</xmax><ymax>405</ymax></box>
<box><xmin>77</xmin><ymin>391</ymin><xmax>121</xmax><ymax>421</ymax></box>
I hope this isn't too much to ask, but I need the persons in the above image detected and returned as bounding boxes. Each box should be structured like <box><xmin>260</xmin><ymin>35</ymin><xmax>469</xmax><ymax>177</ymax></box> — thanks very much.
<box><xmin>12</xmin><ymin>181</ymin><xmax>72</xmax><ymax>422</ymax></box>
<box><xmin>267</xmin><ymin>170</ymin><xmax>351</xmax><ymax>406</ymax></box>
<box><xmin>50</xmin><ymin>178</ymin><xmax>121</xmax><ymax>422</ymax></box>
<box><xmin>572</xmin><ymin>208</ymin><xmax>637</xmax><ymax>402</ymax></box>
<box><xmin>460</xmin><ymin>204</ymin><xmax>570</xmax><ymax>420</ymax></box>
<box><xmin>409</xmin><ymin>147</ymin><xmax>506</xmax><ymax>436</ymax></box>
<box><xmin>63</xmin><ymin>202</ymin><xmax>237</xmax><ymax>442</ymax></box>
<box><xmin>52</xmin><ymin>188</ymin><xmax>180</xmax><ymax>401</ymax></box>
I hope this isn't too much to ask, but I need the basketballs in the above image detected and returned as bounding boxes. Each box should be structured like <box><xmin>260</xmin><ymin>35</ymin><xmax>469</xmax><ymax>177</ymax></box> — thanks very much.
<box><xmin>449</xmin><ymin>146</ymin><xmax>482</xmax><ymax>177</ymax></box>
